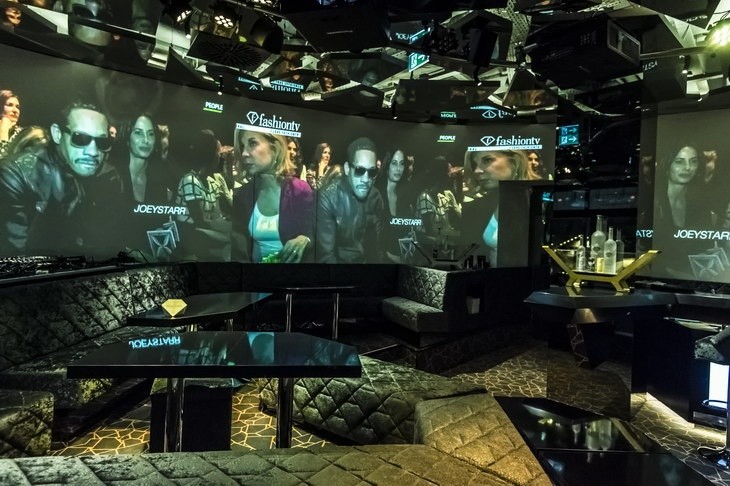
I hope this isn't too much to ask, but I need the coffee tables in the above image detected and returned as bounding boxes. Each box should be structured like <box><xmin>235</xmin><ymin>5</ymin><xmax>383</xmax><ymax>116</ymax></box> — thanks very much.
<box><xmin>271</xmin><ymin>283</ymin><xmax>356</xmax><ymax>342</ymax></box>
<box><xmin>127</xmin><ymin>293</ymin><xmax>276</xmax><ymax>330</ymax></box>
<box><xmin>66</xmin><ymin>330</ymin><xmax>362</xmax><ymax>454</ymax></box>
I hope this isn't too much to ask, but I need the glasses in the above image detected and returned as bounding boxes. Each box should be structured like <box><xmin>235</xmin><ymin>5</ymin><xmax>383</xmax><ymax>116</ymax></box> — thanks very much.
<box><xmin>348</xmin><ymin>163</ymin><xmax>379</xmax><ymax>178</ymax></box>
<box><xmin>63</xmin><ymin>126</ymin><xmax>112</xmax><ymax>151</ymax></box>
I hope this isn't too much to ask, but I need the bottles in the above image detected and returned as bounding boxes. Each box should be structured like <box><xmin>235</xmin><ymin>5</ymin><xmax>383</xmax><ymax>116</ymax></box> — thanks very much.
<box><xmin>536</xmin><ymin>410</ymin><xmax>622</xmax><ymax>464</ymax></box>
<box><xmin>573</xmin><ymin>214</ymin><xmax>625</xmax><ymax>275</ymax></box>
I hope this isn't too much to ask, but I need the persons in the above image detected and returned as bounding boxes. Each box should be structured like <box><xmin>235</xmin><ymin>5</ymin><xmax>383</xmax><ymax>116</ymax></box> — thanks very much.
<box><xmin>655</xmin><ymin>139</ymin><xmax>730</xmax><ymax>283</ymax></box>
<box><xmin>0</xmin><ymin>88</ymin><xmax>549</xmax><ymax>266</ymax></box>
<box><xmin>5</xmin><ymin>0</ymin><xmax>22</xmax><ymax>25</ymax></box>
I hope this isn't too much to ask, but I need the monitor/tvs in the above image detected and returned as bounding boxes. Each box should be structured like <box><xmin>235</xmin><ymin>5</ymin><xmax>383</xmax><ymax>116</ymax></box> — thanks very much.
<box><xmin>637</xmin><ymin>91</ymin><xmax>730</xmax><ymax>292</ymax></box>
<box><xmin>0</xmin><ymin>41</ymin><xmax>563</xmax><ymax>278</ymax></box>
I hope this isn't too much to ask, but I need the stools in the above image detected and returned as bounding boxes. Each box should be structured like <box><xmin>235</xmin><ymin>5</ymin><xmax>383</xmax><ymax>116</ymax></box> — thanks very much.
<box><xmin>0</xmin><ymin>388</ymin><xmax>54</xmax><ymax>458</ymax></box>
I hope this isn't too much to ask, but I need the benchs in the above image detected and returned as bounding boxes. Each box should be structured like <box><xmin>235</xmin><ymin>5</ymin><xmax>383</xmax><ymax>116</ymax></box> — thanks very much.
<box><xmin>259</xmin><ymin>355</ymin><xmax>488</xmax><ymax>443</ymax></box>
<box><xmin>1</xmin><ymin>444</ymin><xmax>518</xmax><ymax>486</ymax></box>
<box><xmin>411</xmin><ymin>390</ymin><xmax>556</xmax><ymax>486</ymax></box>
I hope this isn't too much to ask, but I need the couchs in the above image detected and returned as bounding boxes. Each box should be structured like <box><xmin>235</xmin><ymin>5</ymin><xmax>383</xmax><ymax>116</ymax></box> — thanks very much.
<box><xmin>0</xmin><ymin>265</ymin><xmax>216</xmax><ymax>412</ymax></box>
<box><xmin>196</xmin><ymin>262</ymin><xmax>464</xmax><ymax>336</ymax></box>
